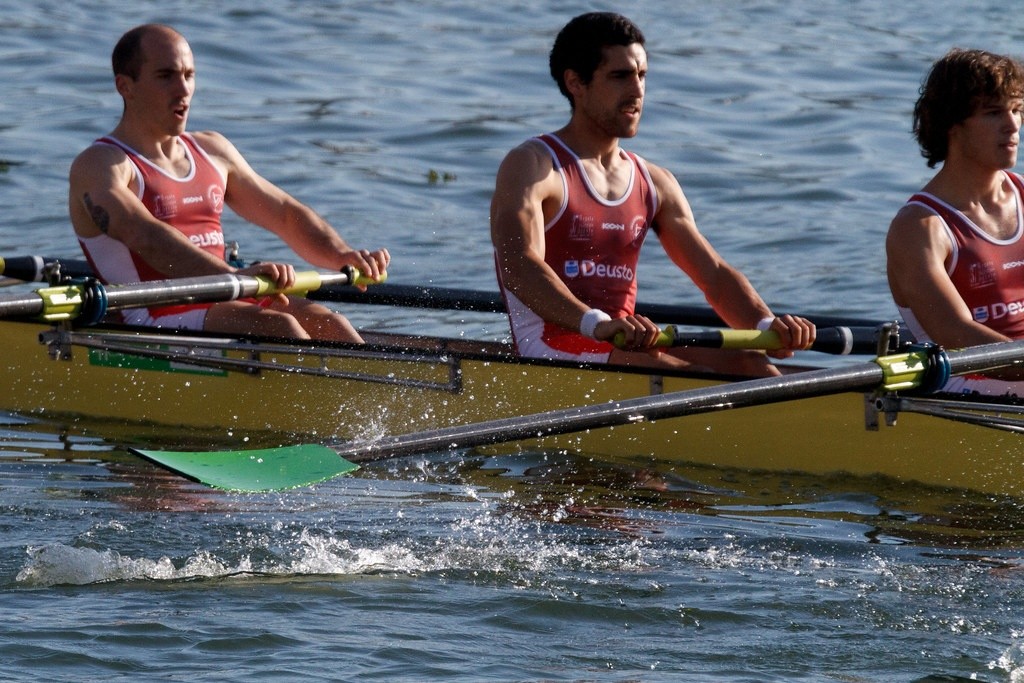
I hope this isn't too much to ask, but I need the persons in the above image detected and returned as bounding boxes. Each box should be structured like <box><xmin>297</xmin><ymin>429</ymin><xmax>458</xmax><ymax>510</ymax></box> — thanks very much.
<box><xmin>490</xmin><ymin>12</ymin><xmax>816</xmax><ymax>379</ymax></box>
<box><xmin>885</xmin><ymin>49</ymin><xmax>1023</xmax><ymax>397</ymax></box>
<box><xmin>68</xmin><ymin>24</ymin><xmax>391</xmax><ymax>344</ymax></box>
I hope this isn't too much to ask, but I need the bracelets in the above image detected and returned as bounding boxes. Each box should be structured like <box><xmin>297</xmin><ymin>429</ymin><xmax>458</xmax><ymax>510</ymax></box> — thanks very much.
<box><xmin>757</xmin><ymin>318</ymin><xmax>775</xmax><ymax>331</ymax></box>
<box><xmin>581</xmin><ymin>308</ymin><xmax>611</xmax><ymax>342</ymax></box>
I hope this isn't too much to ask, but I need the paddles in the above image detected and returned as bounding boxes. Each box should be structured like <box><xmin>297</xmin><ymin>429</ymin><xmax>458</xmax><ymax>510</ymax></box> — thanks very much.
<box><xmin>126</xmin><ymin>340</ymin><xmax>1023</xmax><ymax>492</ymax></box>
<box><xmin>1</xmin><ymin>268</ymin><xmax>389</xmax><ymax>321</ymax></box>
<box><xmin>615</xmin><ymin>314</ymin><xmax>918</xmax><ymax>353</ymax></box>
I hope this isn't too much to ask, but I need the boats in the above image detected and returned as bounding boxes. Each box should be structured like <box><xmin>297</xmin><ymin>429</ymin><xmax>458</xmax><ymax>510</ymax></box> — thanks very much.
<box><xmin>0</xmin><ymin>293</ymin><xmax>1024</xmax><ymax>508</ymax></box>
